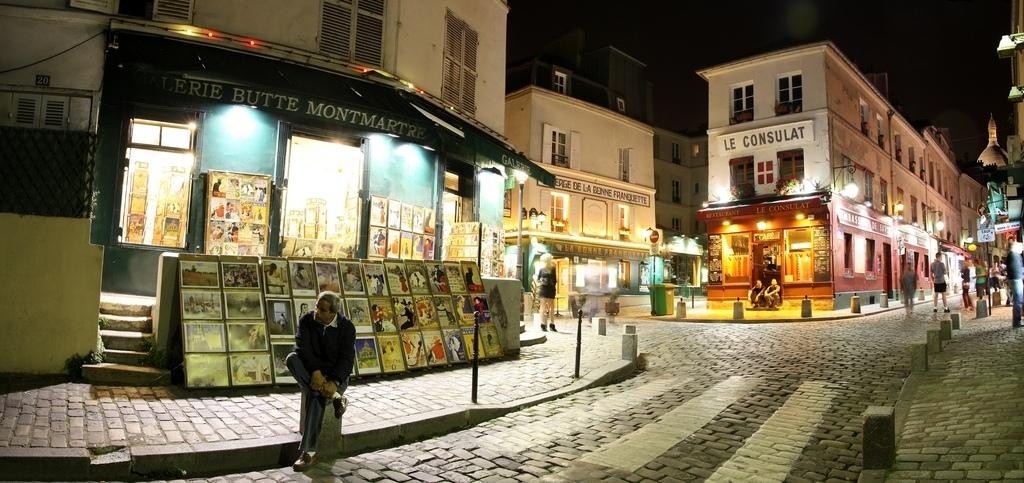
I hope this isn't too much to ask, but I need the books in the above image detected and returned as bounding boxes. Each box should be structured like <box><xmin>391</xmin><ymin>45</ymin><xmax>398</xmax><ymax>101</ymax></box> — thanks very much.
<box><xmin>182</xmin><ymin>259</ymin><xmax>503</xmax><ymax>388</ymax></box>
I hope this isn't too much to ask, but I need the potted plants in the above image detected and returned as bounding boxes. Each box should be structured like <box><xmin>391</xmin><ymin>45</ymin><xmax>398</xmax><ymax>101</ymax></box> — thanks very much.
<box><xmin>603</xmin><ymin>283</ymin><xmax>622</xmax><ymax>316</ymax></box>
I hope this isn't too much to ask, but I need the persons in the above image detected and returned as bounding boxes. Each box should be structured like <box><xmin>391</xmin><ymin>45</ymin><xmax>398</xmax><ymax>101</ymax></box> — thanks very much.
<box><xmin>746</xmin><ymin>278</ymin><xmax>765</xmax><ymax>309</ymax></box>
<box><xmin>763</xmin><ymin>277</ymin><xmax>781</xmax><ymax>311</ymax></box>
<box><xmin>1006</xmin><ymin>240</ymin><xmax>1024</xmax><ymax>328</ymax></box>
<box><xmin>283</xmin><ymin>290</ymin><xmax>356</xmax><ymax>472</ymax></box>
<box><xmin>900</xmin><ymin>261</ymin><xmax>917</xmax><ymax>314</ymax></box>
<box><xmin>958</xmin><ymin>258</ymin><xmax>1014</xmax><ymax>312</ymax></box>
<box><xmin>535</xmin><ymin>253</ymin><xmax>557</xmax><ymax>332</ymax></box>
<box><xmin>929</xmin><ymin>251</ymin><xmax>951</xmax><ymax>313</ymax></box>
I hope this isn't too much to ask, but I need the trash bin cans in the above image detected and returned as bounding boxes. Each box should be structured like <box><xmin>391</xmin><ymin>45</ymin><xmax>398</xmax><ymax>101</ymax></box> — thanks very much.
<box><xmin>648</xmin><ymin>284</ymin><xmax>678</xmax><ymax>316</ymax></box>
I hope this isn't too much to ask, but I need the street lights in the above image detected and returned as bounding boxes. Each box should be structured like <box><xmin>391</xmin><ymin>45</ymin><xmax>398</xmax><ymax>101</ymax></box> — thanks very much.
<box><xmin>511</xmin><ymin>168</ymin><xmax>529</xmax><ymax>322</ymax></box>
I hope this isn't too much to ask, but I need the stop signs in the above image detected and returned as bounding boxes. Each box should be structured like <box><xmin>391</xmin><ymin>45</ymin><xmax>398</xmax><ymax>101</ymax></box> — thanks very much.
<box><xmin>649</xmin><ymin>230</ymin><xmax>659</xmax><ymax>243</ymax></box>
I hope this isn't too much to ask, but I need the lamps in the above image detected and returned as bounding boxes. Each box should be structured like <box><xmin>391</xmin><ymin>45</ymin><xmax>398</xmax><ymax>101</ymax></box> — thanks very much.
<box><xmin>931</xmin><ymin>209</ymin><xmax>945</xmax><ymax>231</ymax></box>
<box><xmin>890</xmin><ymin>199</ymin><xmax>905</xmax><ymax>220</ymax></box>
<box><xmin>995</xmin><ymin>32</ymin><xmax>1023</xmax><ymax>99</ymax></box>
<box><xmin>536</xmin><ymin>209</ymin><xmax>547</xmax><ymax>227</ymax></box>
<box><xmin>960</xmin><ymin>227</ymin><xmax>973</xmax><ymax>244</ymax></box>
<box><xmin>831</xmin><ymin>164</ymin><xmax>860</xmax><ymax>199</ymax></box>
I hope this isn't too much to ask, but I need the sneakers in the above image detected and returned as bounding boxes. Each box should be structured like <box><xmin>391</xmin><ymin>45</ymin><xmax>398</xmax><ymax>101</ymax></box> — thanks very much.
<box><xmin>934</xmin><ymin>308</ymin><xmax>950</xmax><ymax>312</ymax></box>
<box><xmin>541</xmin><ymin>324</ymin><xmax>557</xmax><ymax>331</ymax></box>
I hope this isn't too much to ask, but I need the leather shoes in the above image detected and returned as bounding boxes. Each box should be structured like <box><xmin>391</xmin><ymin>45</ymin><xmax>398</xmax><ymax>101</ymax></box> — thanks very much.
<box><xmin>293</xmin><ymin>453</ymin><xmax>319</xmax><ymax>471</ymax></box>
<box><xmin>333</xmin><ymin>396</ymin><xmax>347</xmax><ymax>418</ymax></box>
